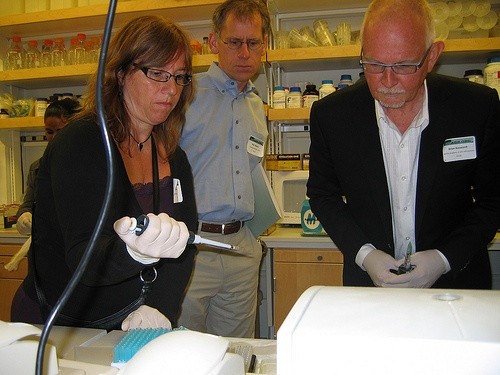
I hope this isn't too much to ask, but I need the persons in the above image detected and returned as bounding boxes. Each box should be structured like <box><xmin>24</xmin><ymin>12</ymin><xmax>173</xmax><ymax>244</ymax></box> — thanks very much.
<box><xmin>306</xmin><ymin>0</ymin><xmax>500</xmax><ymax>290</ymax></box>
<box><xmin>176</xmin><ymin>0</ymin><xmax>270</xmax><ymax>338</ymax></box>
<box><xmin>11</xmin><ymin>14</ymin><xmax>199</xmax><ymax>331</ymax></box>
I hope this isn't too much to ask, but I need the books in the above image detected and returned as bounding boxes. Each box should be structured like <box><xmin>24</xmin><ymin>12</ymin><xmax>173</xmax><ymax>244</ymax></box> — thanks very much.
<box><xmin>244</xmin><ymin>163</ymin><xmax>281</xmax><ymax>238</ymax></box>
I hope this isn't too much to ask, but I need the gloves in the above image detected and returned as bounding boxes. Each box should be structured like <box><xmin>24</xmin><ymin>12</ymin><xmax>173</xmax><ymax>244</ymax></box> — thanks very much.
<box><xmin>113</xmin><ymin>212</ymin><xmax>190</xmax><ymax>264</ymax></box>
<box><xmin>16</xmin><ymin>212</ymin><xmax>32</xmax><ymax>236</ymax></box>
<box><xmin>121</xmin><ymin>305</ymin><xmax>172</xmax><ymax>331</ymax></box>
<box><xmin>362</xmin><ymin>249</ymin><xmax>409</xmax><ymax>288</ymax></box>
<box><xmin>394</xmin><ymin>249</ymin><xmax>446</xmax><ymax>288</ymax></box>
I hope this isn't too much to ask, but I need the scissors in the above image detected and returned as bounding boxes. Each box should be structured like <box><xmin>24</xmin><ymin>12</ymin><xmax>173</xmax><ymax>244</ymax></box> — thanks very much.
<box><xmin>398</xmin><ymin>242</ymin><xmax>415</xmax><ymax>274</ymax></box>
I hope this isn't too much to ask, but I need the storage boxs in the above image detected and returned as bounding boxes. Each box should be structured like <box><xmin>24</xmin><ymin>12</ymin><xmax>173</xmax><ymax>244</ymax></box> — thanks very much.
<box><xmin>267</xmin><ymin>154</ymin><xmax>301</xmax><ymax>171</ymax></box>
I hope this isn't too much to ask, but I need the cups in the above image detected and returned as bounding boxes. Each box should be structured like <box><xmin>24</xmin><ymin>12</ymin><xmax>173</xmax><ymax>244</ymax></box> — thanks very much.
<box><xmin>274</xmin><ymin>19</ymin><xmax>351</xmax><ymax>49</ymax></box>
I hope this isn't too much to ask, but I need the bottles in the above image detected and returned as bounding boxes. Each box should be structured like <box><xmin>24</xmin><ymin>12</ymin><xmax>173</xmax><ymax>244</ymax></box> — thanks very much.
<box><xmin>4</xmin><ymin>30</ymin><xmax>105</xmax><ymax>69</ymax></box>
<box><xmin>462</xmin><ymin>69</ymin><xmax>484</xmax><ymax>85</ymax></box>
<box><xmin>272</xmin><ymin>74</ymin><xmax>354</xmax><ymax>107</ymax></box>
<box><xmin>190</xmin><ymin>36</ymin><xmax>211</xmax><ymax>55</ymax></box>
<box><xmin>34</xmin><ymin>92</ymin><xmax>73</xmax><ymax>117</ymax></box>
<box><xmin>483</xmin><ymin>57</ymin><xmax>500</xmax><ymax>100</ymax></box>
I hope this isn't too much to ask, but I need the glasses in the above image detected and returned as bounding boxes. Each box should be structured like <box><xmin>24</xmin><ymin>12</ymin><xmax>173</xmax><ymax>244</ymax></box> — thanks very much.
<box><xmin>215</xmin><ymin>30</ymin><xmax>264</xmax><ymax>50</ymax></box>
<box><xmin>129</xmin><ymin>61</ymin><xmax>193</xmax><ymax>87</ymax></box>
<box><xmin>359</xmin><ymin>44</ymin><xmax>433</xmax><ymax>76</ymax></box>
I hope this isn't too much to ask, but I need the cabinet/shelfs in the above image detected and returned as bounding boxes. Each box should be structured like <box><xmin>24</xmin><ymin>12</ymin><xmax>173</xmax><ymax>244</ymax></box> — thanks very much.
<box><xmin>0</xmin><ymin>244</ymin><xmax>28</xmax><ymax>322</ymax></box>
<box><xmin>0</xmin><ymin>1</ymin><xmax>271</xmax><ymax>129</ymax></box>
<box><xmin>273</xmin><ymin>248</ymin><xmax>344</xmax><ymax>339</ymax></box>
<box><xmin>267</xmin><ymin>0</ymin><xmax>499</xmax><ymax>170</ymax></box>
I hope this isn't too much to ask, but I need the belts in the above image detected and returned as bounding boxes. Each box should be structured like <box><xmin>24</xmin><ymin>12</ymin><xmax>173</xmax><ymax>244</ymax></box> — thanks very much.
<box><xmin>197</xmin><ymin>220</ymin><xmax>244</xmax><ymax>234</ymax></box>
